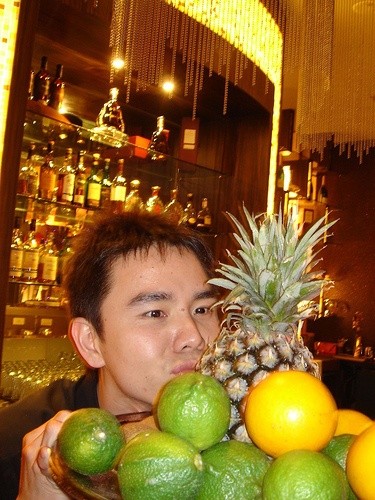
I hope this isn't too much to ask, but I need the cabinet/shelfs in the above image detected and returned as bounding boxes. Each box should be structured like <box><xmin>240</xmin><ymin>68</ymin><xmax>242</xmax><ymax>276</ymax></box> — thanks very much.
<box><xmin>4</xmin><ymin>106</ymin><xmax>225</xmax><ymax>334</ymax></box>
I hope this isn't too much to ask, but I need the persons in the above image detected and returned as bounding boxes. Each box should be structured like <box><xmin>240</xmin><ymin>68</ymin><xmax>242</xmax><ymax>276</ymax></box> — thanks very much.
<box><xmin>0</xmin><ymin>206</ymin><xmax>222</xmax><ymax>500</ymax></box>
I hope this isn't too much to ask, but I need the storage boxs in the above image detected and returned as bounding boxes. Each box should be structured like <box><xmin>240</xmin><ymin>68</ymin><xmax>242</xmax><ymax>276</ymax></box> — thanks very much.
<box><xmin>5</xmin><ymin>305</ymin><xmax>68</xmax><ymax>336</ymax></box>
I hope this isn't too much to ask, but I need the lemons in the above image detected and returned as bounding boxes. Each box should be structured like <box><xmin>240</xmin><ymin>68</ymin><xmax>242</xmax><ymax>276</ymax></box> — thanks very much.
<box><xmin>56</xmin><ymin>370</ymin><xmax>375</xmax><ymax>500</ymax></box>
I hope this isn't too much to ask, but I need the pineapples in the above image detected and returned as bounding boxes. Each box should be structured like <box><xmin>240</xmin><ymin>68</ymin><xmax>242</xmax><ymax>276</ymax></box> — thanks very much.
<box><xmin>195</xmin><ymin>201</ymin><xmax>341</xmax><ymax>449</ymax></box>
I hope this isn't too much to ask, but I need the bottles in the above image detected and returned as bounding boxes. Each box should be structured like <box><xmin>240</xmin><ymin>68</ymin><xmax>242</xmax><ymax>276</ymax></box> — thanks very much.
<box><xmin>9</xmin><ymin>59</ymin><xmax>213</xmax><ymax>287</ymax></box>
<box><xmin>318</xmin><ymin>176</ymin><xmax>327</xmax><ymax>204</ymax></box>
